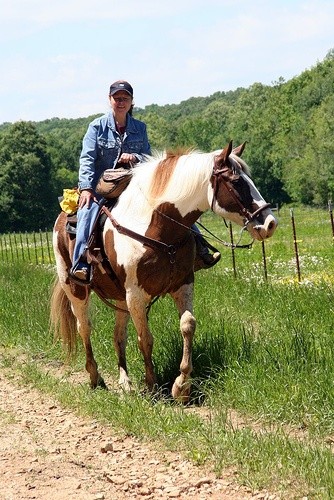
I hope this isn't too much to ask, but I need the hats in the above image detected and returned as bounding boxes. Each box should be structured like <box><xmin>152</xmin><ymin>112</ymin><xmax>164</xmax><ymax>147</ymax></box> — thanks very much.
<box><xmin>109</xmin><ymin>80</ymin><xmax>133</xmax><ymax>97</ymax></box>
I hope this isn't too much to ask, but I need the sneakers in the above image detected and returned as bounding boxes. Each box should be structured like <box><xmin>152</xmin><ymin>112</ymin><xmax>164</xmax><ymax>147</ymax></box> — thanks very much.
<box><xmin>195</xmin><ymin>248</ymin><xmax>221</xmax><ymax>273</ymax></box>
<box><xmin>75</xmin><ymin>263</ymin><xmax>89</xmax><ymax>281</ymax></box>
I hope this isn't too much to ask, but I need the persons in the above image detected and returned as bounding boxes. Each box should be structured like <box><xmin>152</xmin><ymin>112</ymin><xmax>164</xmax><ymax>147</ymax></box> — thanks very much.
<box><xmin>73</xmin><ymin>80</ymin><xmax>151</xmax><ymax>286</ymax></box>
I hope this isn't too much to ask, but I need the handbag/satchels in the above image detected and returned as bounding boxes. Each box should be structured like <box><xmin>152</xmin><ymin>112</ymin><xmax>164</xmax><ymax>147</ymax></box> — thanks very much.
<box><xmin>95</xmin><ymin>168</ymin><xmax>133</xmax><ymax>195</ymax></box>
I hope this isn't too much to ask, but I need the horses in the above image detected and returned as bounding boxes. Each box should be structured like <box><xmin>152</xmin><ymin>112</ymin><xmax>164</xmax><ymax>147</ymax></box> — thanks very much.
<box><xmin>47</xmin><ymin>139</ymin><xmax>277</xmax><ymax>403</ymax></box>
